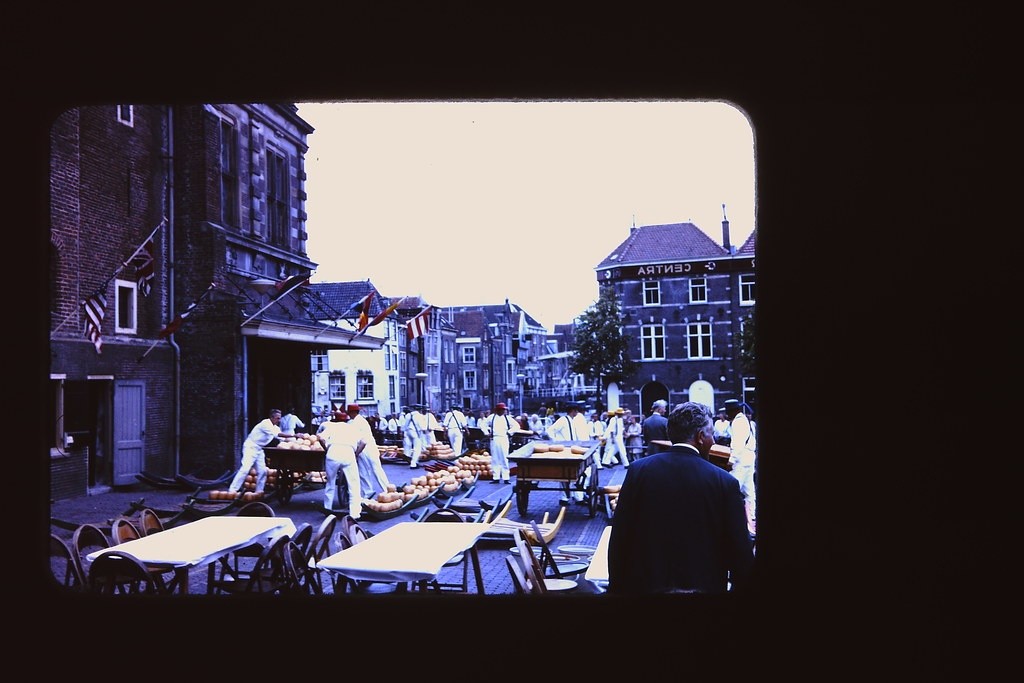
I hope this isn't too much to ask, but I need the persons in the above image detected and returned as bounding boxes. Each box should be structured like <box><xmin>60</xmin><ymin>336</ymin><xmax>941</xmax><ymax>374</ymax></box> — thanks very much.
<box><xmin>607</xmin><ymin>403</ymin><xmax>757</xmax><ymax>594</ymax></box>
<box><xmin>228</xmin><ymin>402</ymin><xmax>641</xmax><ymax>518</ymax></box>
<box><xmin>643</xmin><ymin>399</ymin><xmax>757</xmax><ymax>540</ymax></box>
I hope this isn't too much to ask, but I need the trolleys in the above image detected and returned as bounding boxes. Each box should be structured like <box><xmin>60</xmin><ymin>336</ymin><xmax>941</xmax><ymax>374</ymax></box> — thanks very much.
<box><xmin>379</xmin><ymin>426</ymin><xmax>535</xmax><ymax>454</ymax></box>
<box><xmin>260</xmin><ymin>448</ymin><xmax>351</xmax><ymax>508</ymax></box>
<box><xmin>503</xmin><ymin>436</ymin><xmax>603</xmax><ymax>519</ymax></box>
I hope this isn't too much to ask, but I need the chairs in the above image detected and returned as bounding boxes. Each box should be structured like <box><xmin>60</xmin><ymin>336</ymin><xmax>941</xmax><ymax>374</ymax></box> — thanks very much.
<box><xmin>49</xmin><ymin>509</ymin><xmax>590</xmax><ymax>599</ymax></box>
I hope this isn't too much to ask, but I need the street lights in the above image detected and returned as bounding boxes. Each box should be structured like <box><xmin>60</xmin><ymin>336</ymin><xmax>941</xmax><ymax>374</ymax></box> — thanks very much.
<box><xmin>516</xmin><ymin>372</ymin><xmax>525</xmax><ymax>415</ymax></box>
<box><xmin>415</xmin><ymin>373</ymin><xmax>428</xmax><ymax>416</ymax></box>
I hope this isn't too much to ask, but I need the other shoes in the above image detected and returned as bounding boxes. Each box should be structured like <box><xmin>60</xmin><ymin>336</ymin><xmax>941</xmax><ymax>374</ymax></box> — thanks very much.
<box><xmin>410</xmin><ymin>466</ymin><xmax>420</xmax><ymax>469</ymax></box>
<box><xmin>559</xmin><ymin>500</ymin><xmax>571</xmax><ymax>506</ymax></box>
<box><xmin>489</xmin><ymin>480</ymin><xmax>500</xmax><ymax>484</ymax></box>
<box><xmin>575</xmin><ymin>500</ymin><xmax>587</xmax><ymax>505</ymax></box>
<box><xmin>602</xmin><ymin>463</ymin><xmax>613</xmax><ymax>469</ymax></box>
<box><xmin>624</xmin><ymin>466</ymin><xmax>628</xmax><ymax>469</ymax></box>
<box><xmin>504</xmin><ymin>480</ymin><xmax>511</xmax><ymax>484</ymax></box>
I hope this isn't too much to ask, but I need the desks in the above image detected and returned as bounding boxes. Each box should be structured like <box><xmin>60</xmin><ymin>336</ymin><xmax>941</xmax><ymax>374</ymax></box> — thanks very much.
<box><xmin>586</xmin><ymin>526</ymin><xmax>613</xmax><ymax>589</ymax></box>
<box><xmin>87</xmin><ymin>515</ymin><xmax>297</xmax><ymax>593</ymax></box>
<box><xmin>317</xmin><ymin>521</ymin><xmax>487</xmax><ymax>594</ymax></box>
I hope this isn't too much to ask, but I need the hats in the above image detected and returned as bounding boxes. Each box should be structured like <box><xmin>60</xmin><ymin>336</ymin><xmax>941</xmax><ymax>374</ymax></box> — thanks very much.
<box><xmin>719</xmin><ymin>398</ymin><xmax>745</xmax><ymax>411</ymax></box>
<box><xmin>493</xmin><ymin>403</ymin><xmax>508</xmax><ymax>410</ymax></box>
<box><xmin>348</xmin><ymin>404</ymin><xmax>360</xmax><ymax>411</ymax></box>
<box><xmin>565</xmin><ymin>401</ymin><xmax>578</xmax><ymax>407</ymax></box>
<box><xmin>576</xmin><ymin>400</ymin><xmax>591</xmax><ymax>409</ymax></box>
<box><xmin>334</xmin><ymin>412</ymin><xmax>347</xmax><ymax>420</ymax></box>
<box><xmin>608</xmin><ymin>411</ymin><xmax>615</xmax><ymax>416</ymax></box>
<box><xmin>615</xmin><ymin>408</ymin><xmax>625</xmax><ymax>414</ymax></box>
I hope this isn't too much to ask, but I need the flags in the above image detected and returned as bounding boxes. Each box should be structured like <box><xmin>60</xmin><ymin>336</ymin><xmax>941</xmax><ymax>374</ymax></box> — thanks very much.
<box><xmin>82</xmin><ymin>281</ymin><xmax>106</xmax><ymax>354</ymax></box>
<box><xmin>352</xmin><ymin>292</ymin><xmax>373</xmax><ymax>331</ymax></box>
<box><xmin>407</xmin><ymin>309</ymin><xmax>431</xmax><ymax>340</ymax></box>
<box><xmin>362</xmin><ymin>303</ymin><xmax>398</xmax><ymax>335</ymax></box>
<box><xmin>133</xmin><ymin>228</ymin><xmax>157</xmax><ymax>296</ymax></box>
<box><xmin>159</xmin><ymin>289</ymin><xmax>206</xmax><ymax>340</ymax></box>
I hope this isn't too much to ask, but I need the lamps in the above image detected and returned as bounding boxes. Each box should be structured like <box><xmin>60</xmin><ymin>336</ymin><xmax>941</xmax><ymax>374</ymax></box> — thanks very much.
<box><xmin>252</xmin><ymin>279</ymin><xmax>276</xmax><ymax>295</ymax></box>
